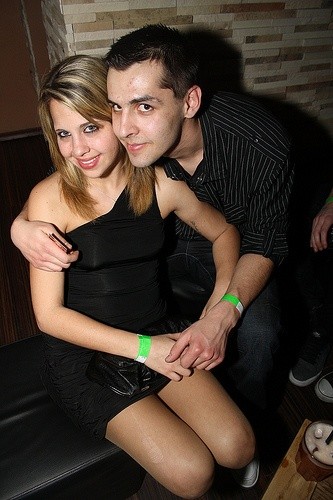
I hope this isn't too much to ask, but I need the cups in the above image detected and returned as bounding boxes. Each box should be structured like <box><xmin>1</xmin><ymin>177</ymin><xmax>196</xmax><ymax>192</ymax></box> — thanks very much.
<box><xmin>295</xmin><ymin>419</ymin><xmax>333</xmax><ymax>482</ymax></box>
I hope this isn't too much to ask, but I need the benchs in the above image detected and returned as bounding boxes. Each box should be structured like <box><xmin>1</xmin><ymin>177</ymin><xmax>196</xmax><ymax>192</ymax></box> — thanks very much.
<box><xmin>0</xmin><ymin>335</ymin><xmax>142</xmax><ymax>500</ymax></box>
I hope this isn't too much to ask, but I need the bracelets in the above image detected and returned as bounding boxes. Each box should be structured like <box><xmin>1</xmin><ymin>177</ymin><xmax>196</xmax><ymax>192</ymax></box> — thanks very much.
<box><xmin>221</xmin><ymin>294</ymin><xmax>243</xmax><ymax>317</ymax></box>
<box><xmin>135</xmin><ymin>334</ymin><xmax>151</xmax><ymax>364</ymax></box>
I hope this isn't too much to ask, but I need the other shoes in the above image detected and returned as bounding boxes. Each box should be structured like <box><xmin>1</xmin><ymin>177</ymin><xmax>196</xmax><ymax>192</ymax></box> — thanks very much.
<box><xmin>232</xmin><ymin>453</ymin><xmax>258</xmax><ymax>487</ymax></box>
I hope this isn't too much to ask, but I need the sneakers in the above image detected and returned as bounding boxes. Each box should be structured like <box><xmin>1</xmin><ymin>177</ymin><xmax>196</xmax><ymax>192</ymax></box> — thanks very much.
<box><xmin>315</xmin><ymin>370</ymin><xmax>333</xmax><ymax>402</ymax></box>
<box><xmin>289</xmin><ymin>334</ymin><xmax>330</xmax><ymax>387</ymax></box>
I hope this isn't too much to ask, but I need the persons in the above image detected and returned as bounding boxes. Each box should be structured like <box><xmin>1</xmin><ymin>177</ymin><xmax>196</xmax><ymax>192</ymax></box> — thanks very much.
<box><xmin>28</xmin><ymin>55</ymin><xmax>254</xmax><ymax>499</ymax></box>
<box><xmin>289</xmin><ymin>193</ymin><xmax>333</xmax><ymax>403</ymax></box>
<box><xmin>11</xmin><ymin>24</ymin><xmax>293</xmax><ymax>488</ymax></box>
